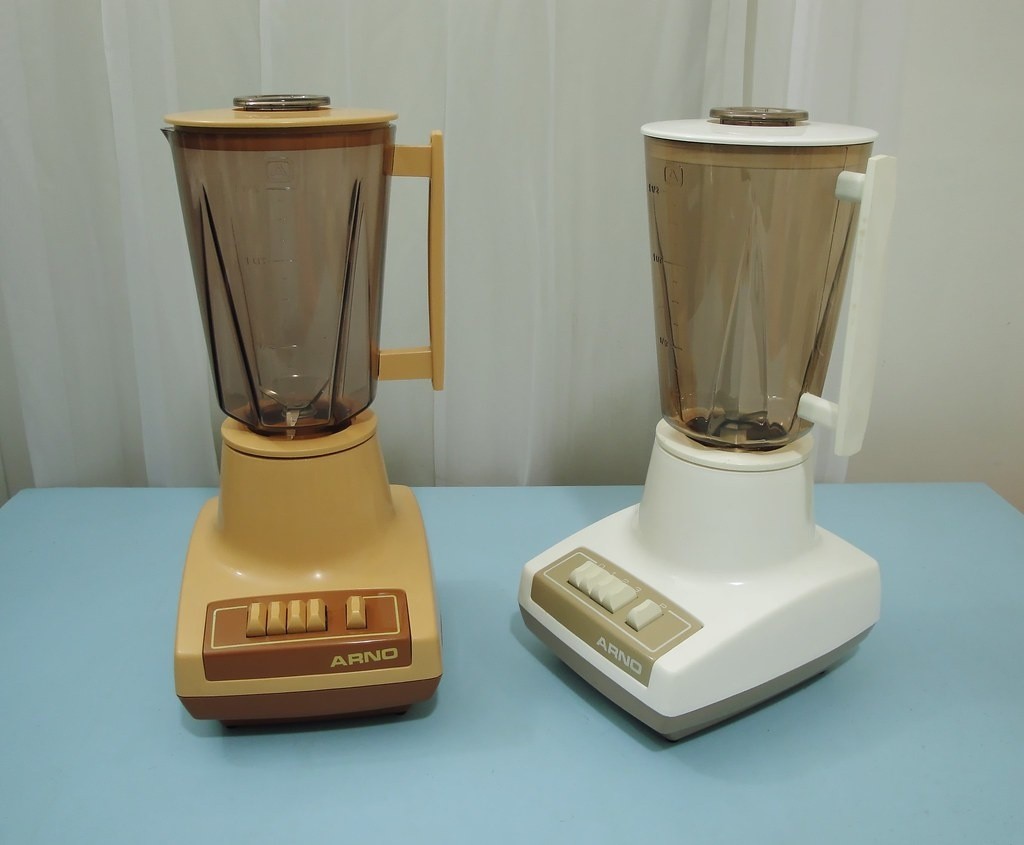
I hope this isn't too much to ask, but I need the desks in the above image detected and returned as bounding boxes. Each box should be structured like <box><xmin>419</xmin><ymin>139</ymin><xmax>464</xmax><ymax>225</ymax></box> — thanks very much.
<box><xmin>0</xmin><ymin>485</ymin><xmax>1024</xmax><ymax>845</ymax></box>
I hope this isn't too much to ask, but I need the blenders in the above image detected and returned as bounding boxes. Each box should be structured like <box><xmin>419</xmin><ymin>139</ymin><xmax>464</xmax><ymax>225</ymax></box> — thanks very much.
<box><xmin>159</xmin><ymin>97</ymin><xmax>444</xmax><ymax>719</ymax></box>
<box><xmin>519</xmin><ymin>108</ymin><xmax>897</xmax><ymax>741</ymax></box>
